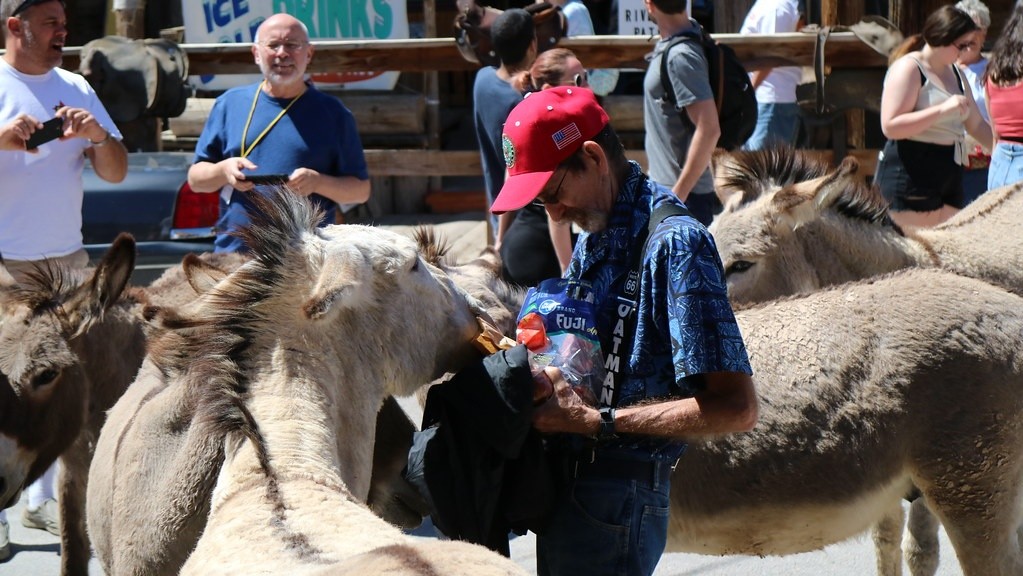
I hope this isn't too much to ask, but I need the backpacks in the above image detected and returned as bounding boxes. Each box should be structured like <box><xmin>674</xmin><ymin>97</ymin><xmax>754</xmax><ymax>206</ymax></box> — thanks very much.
<box><xmin>660</xmin><ymin>31</ymin><xmax>758</xmax><ymax>153</ymax></box>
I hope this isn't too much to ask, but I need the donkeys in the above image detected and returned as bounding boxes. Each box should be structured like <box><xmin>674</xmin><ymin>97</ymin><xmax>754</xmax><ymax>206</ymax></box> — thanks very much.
<box><xmin>0</xmin><ymin>177</ymin><xmax>532</xmax><ymax>576</ymax></box>
<box><xmin>706</xmin><ymin>132</ymin><xmax>1023</xmax><ymax>576</ymax></box>
<box><xmin>413</xmin><ymin>213</ymin><xmax>1023</xmax><ymax>576</ymax></box>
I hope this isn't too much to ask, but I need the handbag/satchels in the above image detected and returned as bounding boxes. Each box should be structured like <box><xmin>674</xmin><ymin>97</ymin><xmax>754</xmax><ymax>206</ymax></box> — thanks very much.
<box><xmin>498</xmin><ymin>424</ymin><xmax>596</xmax><ymax>538</ymax></box>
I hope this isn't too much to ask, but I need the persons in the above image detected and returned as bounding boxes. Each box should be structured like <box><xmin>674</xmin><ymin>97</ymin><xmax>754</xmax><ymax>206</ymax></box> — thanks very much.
<box><xmin>737</xmin><ymin>0</ymin><xmax>823</xmax><ymax>152</ymax></box>
<box><xmin>188</xmin><ymin>13</ymin><xmax>372</xmax><ymax>253</ymax></box>
<box><xmin>953</xmin><ymin>0</ymin><xmax>1023</xmax><ymax>203</ymax></box>
<box><xmin>489</xmin><ymin>86</ymin><xmax>759</xmax><ymax>576</ymax></box>
<box><xmin>873</xmin><ymin>4</ymin><xmax>993</xmax><ymax>230</ymax></box>
<box><xmin>643</xmin><ymin>0</ymin><xmax>725</xmax><ymax>227</ymax></box>
<box><xmin>474</xmin><ymin>0</ymin><xmax>596</xmax><ymax>289</ymax></box>
<box><xmin>0</xmin><ymin>0</ymin><xmax>131</xmax><ymax>562</ymax></box>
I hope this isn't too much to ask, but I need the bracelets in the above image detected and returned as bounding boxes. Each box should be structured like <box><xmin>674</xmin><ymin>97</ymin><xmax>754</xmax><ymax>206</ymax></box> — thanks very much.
<box><xmin>90</xmin><ymin>131</ymin><xmax>111</xmax><ymax>147</ymax></box>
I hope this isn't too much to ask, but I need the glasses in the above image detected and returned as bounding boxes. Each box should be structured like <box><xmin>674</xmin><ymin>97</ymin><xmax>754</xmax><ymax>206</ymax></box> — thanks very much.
<box><xmin>549</xmin><ymin>72</ymin><xmax>588</xmax><ymax>87</ymax></box>
<box><xmin>11</xmin><ymin>0</ymin><xmax>66</xmax><ymax>17</ymax></box>
<box><xmin>533</xmin><ymin>168</ymin><xmax>568</xmax><ymax>206</ymax></box>
<box><xmin>952</xmin><ymin>41</ymin><xmax>976</xmax><ymax>51</ymax></box>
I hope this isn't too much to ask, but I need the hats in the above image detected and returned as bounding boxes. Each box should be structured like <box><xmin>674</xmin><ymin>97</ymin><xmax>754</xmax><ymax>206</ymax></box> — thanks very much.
<box><xmin>489</xmin><ymin>85</ymin><xmax>609</xmax><ymax>215</ymax></box>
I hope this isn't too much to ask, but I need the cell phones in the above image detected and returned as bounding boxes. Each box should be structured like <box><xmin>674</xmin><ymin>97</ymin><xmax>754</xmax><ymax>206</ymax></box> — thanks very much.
<box><xmin>26</xmin><ymin>117</ymin><xmax>65</xmax><ymax>150</ymax></box>
<box><xmin>243</xmin><ymin>174</ymin><xmax>289</xmax><ymax>185</ymax></box>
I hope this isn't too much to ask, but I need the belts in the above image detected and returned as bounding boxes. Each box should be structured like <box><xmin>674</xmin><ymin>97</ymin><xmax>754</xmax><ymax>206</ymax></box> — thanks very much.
<box><xmin>574</xmin><ymin>455</ymin><xmax>652</xmax><ymax>474</ymax></box>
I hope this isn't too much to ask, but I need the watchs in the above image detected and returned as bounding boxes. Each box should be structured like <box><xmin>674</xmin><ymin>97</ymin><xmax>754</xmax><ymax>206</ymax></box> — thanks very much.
<box><xmin>598</xmin><ymin>408</ymin><xmax>619</xmax><ymax>440</ymax></box>
